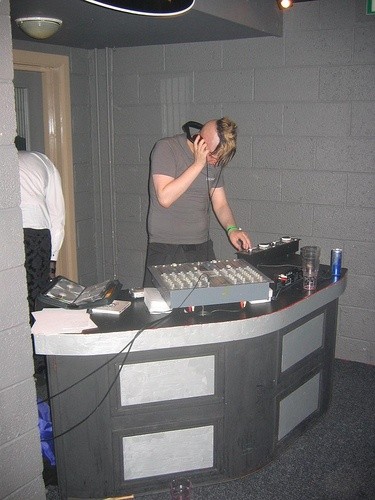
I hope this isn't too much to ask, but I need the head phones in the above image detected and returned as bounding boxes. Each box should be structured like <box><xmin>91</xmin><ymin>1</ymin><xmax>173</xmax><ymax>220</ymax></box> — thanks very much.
<box><xmin>181</xmin><ymin>120</ymin><xmax>205</xmax><ymax>144</ymax></box>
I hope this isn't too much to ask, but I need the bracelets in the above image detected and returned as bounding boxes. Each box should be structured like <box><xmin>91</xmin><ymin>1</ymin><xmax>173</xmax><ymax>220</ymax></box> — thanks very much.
<box><xmin>225</xmin><ymin>226</ymin><xmax>243</xmax><ymax>232</ymax></box>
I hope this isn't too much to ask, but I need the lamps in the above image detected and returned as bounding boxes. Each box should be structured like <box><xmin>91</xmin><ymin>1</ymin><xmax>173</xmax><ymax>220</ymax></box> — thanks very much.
<box><xmin>13</xmin><ymin>18</ymin><xmax>64</xmax><ymax>40</ymax></box>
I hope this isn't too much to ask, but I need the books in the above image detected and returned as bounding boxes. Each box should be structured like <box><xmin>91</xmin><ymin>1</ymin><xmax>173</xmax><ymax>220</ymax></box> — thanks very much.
<box><xmin>91</xmin><ymin>299</ymin><xmax>132</xmax><ymax>315</ymax></box>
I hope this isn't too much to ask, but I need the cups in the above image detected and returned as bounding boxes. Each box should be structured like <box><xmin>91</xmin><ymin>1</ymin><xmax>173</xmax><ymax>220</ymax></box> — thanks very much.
<box><xmin>301</xmin><ymin>245</ymin><xmax>321</xmax><ymax>290</ymax></box>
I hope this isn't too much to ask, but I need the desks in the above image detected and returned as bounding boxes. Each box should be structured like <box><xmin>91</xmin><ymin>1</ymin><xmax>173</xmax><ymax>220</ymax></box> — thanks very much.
<box><xmin>34</xmin><ymin>264</ymin><xmax>348</xmax><ymax>500</ymax></box>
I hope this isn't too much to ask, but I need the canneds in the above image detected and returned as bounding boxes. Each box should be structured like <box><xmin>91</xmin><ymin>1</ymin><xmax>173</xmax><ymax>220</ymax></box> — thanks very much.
<box><xmin>330</xmin><ymin>248</ymin><xmax>343</xmax><ymax>276</ymax></box>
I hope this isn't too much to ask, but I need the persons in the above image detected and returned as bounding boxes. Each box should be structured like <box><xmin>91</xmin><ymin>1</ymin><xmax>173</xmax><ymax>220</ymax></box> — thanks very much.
<box><xmin>142</xmin><ymin>118</ymin><xmax>252</xmax><ymax>289</ymax></box>
<box><xmin>15</xmin><ymin>136</ymin><xmax>66</xmax><ymax>374</ymax></box>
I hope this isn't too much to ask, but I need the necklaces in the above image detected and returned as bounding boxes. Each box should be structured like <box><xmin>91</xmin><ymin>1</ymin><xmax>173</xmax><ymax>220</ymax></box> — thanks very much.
<box><xmin>205</xmin><ymin>159</ymin><xmax>224</xmax><ymax>215</ymax></box>
